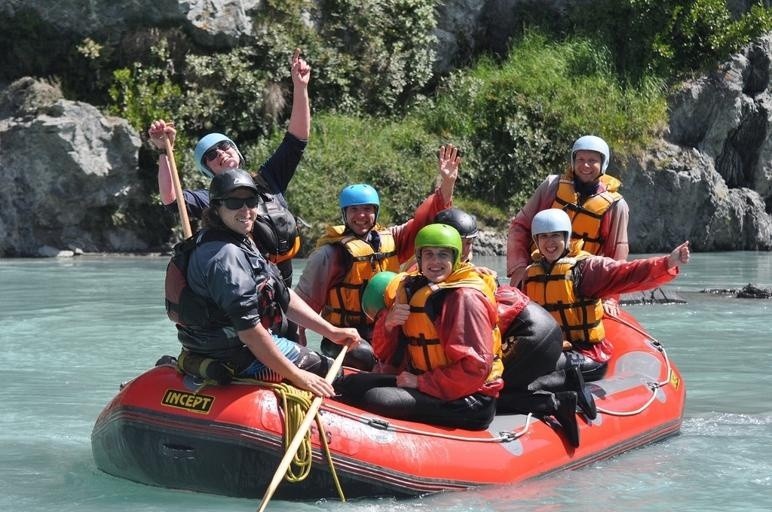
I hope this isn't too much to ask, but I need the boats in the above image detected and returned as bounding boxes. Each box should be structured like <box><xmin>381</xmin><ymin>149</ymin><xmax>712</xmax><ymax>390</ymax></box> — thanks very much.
<box><xmin>90</xmin><ymin>303</ymin><xmax>686</xmax><ymax>500</ymax></box>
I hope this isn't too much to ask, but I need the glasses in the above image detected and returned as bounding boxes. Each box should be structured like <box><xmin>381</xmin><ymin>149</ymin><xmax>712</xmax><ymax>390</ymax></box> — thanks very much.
<box><xmin>206</xmin><ymin>141</ymin><xmax>232</xmax><ymax>161</ymax></box>
<box><xmin>224</xmin><ymin>197</ymin><xmax>259</xmax><ymax>209</ymax></box>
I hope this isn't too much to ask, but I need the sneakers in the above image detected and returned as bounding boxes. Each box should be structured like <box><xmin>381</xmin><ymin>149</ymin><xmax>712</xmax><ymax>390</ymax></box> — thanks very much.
<box><xmin>553</xmin><ymin>365</ymin><xmax>596</xmax><ymax>447</ymax></box>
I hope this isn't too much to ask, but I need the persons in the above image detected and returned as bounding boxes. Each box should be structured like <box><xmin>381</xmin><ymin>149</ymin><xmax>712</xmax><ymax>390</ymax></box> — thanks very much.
<box><xmin>292</xmin><ymin>141</ymin><xmax>463</xmax><ymax>370</ymax></box>
<box><xmin>505</xmin><ymin>134</ymin><xmax>630</xmax><ymax>319</ymax></box>
<box><xmin>175</xmin><ymin>168</ymin><xmax>360</xmax><ymax>400</ymax></box>
<box><xmin>515</xmin><ymin>206</ymin><xmax>691</xmax><ymax>380</ymax></box>
<box><xmin>148</xmin><ymin>48</ymin><xmax>312</xmax><ymax>288</ymax></box>
<box><xmin>359</xmin><ymin>267</ymin><xmax>598</xmax><ymax>448</ymax></box>
<box><xmin>332</xmin><ymin>223</ymin><xmax>500</xmax><ymax>432</ymax></box>
<box><xmin>402</xmin><ymin>207</ymin><xmax>501</xmax><ymax>299</ymax></box>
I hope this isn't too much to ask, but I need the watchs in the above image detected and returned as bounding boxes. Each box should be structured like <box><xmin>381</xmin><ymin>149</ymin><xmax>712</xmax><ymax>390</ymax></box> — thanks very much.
<box><xmin>155</xmin><ymin>147</ymin><xmax>170</xmax><ymax>155</ymax></box>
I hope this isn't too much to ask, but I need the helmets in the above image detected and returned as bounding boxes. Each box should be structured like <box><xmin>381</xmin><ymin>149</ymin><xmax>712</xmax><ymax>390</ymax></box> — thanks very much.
<box><xmin>339</xmin><ymin>183</ymin><xmax>379</xmax><ymax>221</ymax></box>
<box><xmin>193</xmin><ymin>132</ymin><xmax>245</xmax><ymax>178</ymax></box>
<box><xmin>571</xmin><ymin>135</ymin><xmax>610</xmax><ymax>176</ymax></box>
<box><xmin>361</xmin><ymin>271</ymin><xmax>400</xmax><ymax>320</ymax></box>
<box><xmin>414</xmin><ymin>209</ymin><xmax>479</xmax><ymax>270</ymax></box>
<box><xmin>209</xmin><ymin>168</ymin><xmax>259</xmax><ymax>202</ymax></box>
<box><xmin>531</xmin><ymin>209</ymin><xmax>572</xmax><ymax>249</ymax></box>
